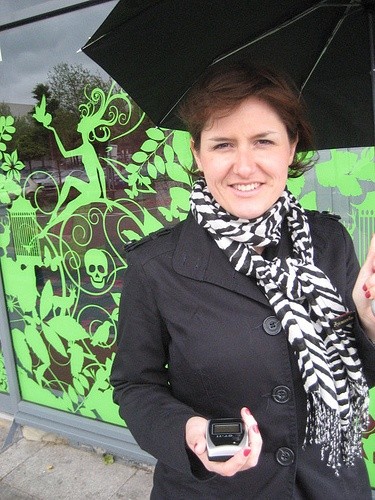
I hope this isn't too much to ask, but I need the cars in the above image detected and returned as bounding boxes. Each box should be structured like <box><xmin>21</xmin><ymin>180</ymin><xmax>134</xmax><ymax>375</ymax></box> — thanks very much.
<box><xmin>32</xmin><ymin>168</ymin><xmax>127</xmax><ymax>188</ymax></box>
<box><xmin>9</xmin><ymin>176</ymin><xmax>39</xmax><ymax>204</ymax></box>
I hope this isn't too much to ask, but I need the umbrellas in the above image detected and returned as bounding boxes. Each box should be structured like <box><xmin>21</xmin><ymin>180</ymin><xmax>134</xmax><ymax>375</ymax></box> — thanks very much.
<box><xmin>75</xmin><ymin>0</ymin><xmax>375</xmax><ymax>153</ymax></box>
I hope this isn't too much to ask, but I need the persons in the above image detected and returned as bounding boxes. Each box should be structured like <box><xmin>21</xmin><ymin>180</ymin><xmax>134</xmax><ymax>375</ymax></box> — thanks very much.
<box><xmin>110</xmin><ymin>64</ymin><xmax>375</xmax><ymax>499</ymax></box>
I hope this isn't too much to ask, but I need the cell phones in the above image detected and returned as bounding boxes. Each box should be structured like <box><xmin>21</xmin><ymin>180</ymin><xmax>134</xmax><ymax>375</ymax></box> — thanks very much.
<box><xmin>206</xmin><ymin>417</ymin><xmax>247</xmax><ymax>461</ymax></box>
